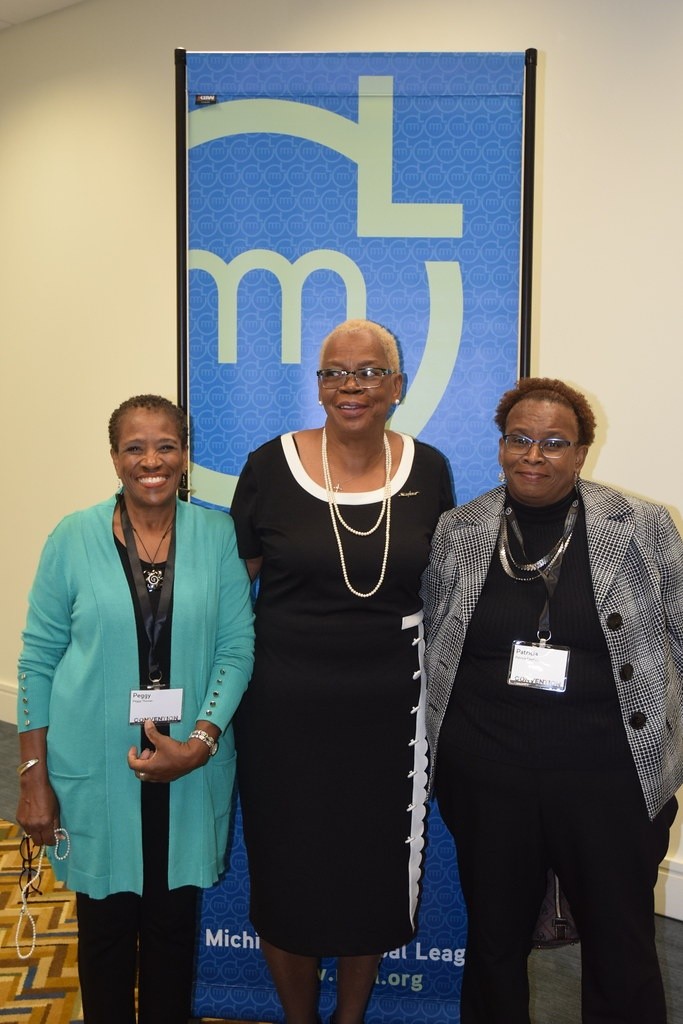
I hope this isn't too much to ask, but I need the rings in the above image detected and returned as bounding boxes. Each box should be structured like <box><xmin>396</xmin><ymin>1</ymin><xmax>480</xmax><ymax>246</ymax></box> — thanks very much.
<box><xmin>140</xmin><ymin>773</ymin><xmax>145</xmax><ymax>780</ymax></box>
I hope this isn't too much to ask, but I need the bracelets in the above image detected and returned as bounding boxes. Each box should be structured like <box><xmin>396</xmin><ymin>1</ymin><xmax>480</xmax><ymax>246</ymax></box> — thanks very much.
<box><xmin>17</xmin><ymin>759</ymin><xmax>38</xmax><ymax>775</ymax></box>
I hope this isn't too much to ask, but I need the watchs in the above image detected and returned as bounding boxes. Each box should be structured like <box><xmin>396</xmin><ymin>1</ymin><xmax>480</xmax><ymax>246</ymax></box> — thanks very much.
<box><xmin>189</xmin><ymin>730</ymin><xmax>218</xmax><ymax>756</ymax></box>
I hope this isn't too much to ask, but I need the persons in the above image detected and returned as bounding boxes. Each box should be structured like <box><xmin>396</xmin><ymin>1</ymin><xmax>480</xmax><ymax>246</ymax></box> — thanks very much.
<box><xmin>16</xmin><ymin>395</ymin><xmax>255</xmax><ymax>1024</ymax></box>
<box><xmin>230</xmin><ymin>321</ymin><xmax>456</xmax><ymax>1024</ymax></box>
<box><xmin>418</xmin><ymin>378</ymin><xmax>683</xmax><ymax>1024</ymax></box>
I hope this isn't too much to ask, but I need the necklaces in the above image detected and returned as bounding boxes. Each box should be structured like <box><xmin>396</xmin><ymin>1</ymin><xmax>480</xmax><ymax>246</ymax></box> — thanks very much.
<box><xmin>497</xmin><ymin>513</ymin><xmax>573</xmax><ymax>581</ymax></box>
<box><xmin>323</xmin><ymin>427</ymin><xmax>392</xmax><ymax>597</ymax></box>
<box><xmin>16</xmin><ymin>828</ymin><xmax>70</xmax><ymax>959</ymax></box>
<box><xmin>333</xmin><ymin>447</ymin><xmax>384</xmax><ymax>493</ymax></box>
<box><xmin>132</xmin><ymin>519</ymin><xmax>174</xmax><ymax>593</ymax></box>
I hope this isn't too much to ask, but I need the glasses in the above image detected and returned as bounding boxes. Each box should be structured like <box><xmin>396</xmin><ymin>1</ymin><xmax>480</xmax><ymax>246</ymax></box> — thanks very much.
<box><xmin>316</xmin><ymin>367</ymin><xmax>396</xmax><ymax>389</ymax></box>
<box><xmin>18</xmin><ymin>835</ymin><xmax>43</xmax><ymax>898</ymax></box>
<box><xmin>502</xmin><ymin>434</ymin><xmax>577</xmax><ymax>458</ymax></box>
